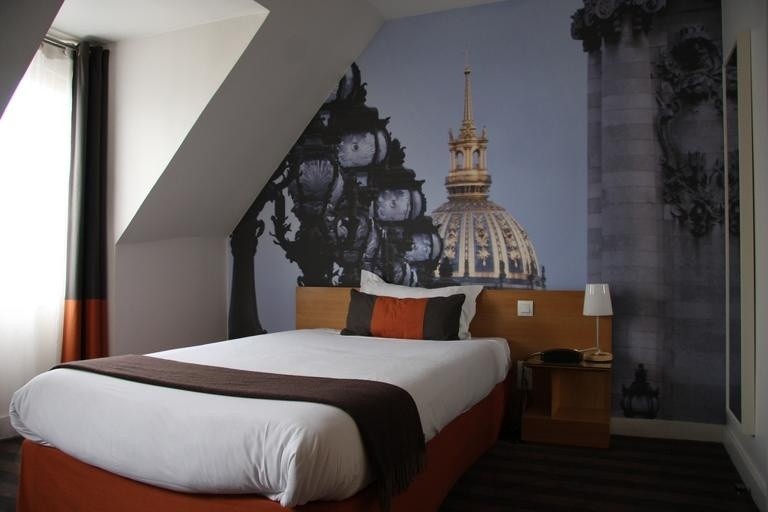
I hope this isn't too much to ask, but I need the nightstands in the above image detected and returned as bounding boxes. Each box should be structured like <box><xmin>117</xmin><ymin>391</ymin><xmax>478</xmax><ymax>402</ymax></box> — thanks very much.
<box><xmin>521</xmin><ymin>361</ymin><xmax>616</xmax><ymax>449</ymax></box>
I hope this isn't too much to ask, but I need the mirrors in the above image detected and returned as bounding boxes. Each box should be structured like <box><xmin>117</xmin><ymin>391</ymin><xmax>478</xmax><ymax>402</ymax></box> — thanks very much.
<box><xmin>721</xmin><ymin>31</ymin><xmax>756</xmax><ymax>437</ymax></box>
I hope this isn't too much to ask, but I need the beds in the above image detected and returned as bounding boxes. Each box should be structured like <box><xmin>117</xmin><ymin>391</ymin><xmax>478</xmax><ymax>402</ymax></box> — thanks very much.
<box><xmin>9</xmin><ymin>286</ymin><xmax>614</xmax><ymax>512</ymax></box>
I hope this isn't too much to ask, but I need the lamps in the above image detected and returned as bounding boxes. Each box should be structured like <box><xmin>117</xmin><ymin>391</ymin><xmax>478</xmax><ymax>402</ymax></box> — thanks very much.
<box><xmin>583</xmin><ymin>283</ymin><xmax>614</xmax><ymax>362</ymax></box>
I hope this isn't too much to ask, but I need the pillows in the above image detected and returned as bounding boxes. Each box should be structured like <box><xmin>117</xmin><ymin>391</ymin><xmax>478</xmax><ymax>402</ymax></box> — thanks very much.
<box><xmin>340</xmin><ymin>288</ymin><xmax>465</xmax><ymax>340</ymax></box>
<box><xmin>360</xmin><ymin>269</ymin><xmax>484</xmax><ymax>340</ymax></box>
